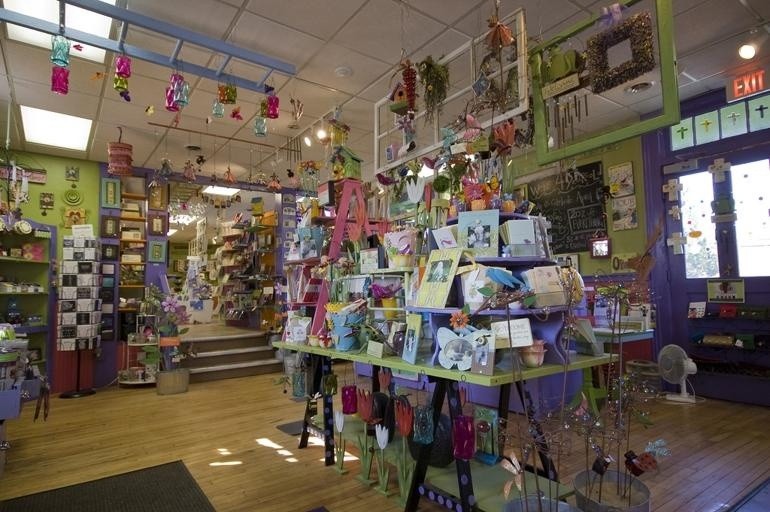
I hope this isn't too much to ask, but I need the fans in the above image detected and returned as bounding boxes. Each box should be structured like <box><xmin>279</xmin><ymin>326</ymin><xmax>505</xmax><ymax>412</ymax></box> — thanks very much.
<box><xmin>657</xmin><ymin>344</ymin><xmax>697</xmax><ymax>404</ymax></box>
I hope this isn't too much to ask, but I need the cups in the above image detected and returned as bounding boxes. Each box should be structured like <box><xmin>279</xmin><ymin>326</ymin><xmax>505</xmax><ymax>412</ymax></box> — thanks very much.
<box><xmin>523</xmin><ymin>340</ymin><xmax>546</xmax><ymax>350</ymax></box>
<box><xmin>307</xmin><ymin>335</ymin><xmax>319</xmax><ymax>346</ymax></box>
<box><xmin>518</xmin><ymin>348</ymin><xmax>547</xmax><ymax>368</ymax></box>
<box><xmin>318</xmin><ymin>335</ymin><xmax>333</xmax><ymax>348</ymax></box>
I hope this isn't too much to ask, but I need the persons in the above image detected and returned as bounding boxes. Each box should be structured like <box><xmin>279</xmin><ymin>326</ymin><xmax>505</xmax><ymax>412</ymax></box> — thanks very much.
<box><xmin>407</xmin><ymin>330</ymin><xmax>414</xmax><ymax>352</ymax></box>
<box><xmin>478</xmin><ymin>348</ymin><xmax>485</xmax><ymax>363</ymax></box>
<box><xmin>472</xmin><ymin>226</ymin><xmax>485</xmax><ymax>248</ymax></box>
<box><xmin>300</xmin><ymin>229</ymin><xmax>316</xmax><ymax>258</ymax></box>
<box><xmin>428</xmin><ymin>261</ymin><xmax>443</xmax><ymax>282</ymax></box>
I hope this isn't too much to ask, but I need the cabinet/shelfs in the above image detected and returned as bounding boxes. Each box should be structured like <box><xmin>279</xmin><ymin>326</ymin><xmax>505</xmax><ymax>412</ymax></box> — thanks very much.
<box><xmin>118</xmin><ymin>192</ymin><xmax>146</xmax><ymax>311</ymax></box>
<box><xmin>0</xmin><ymin>216</ymin><xmax>57</xmax><ymax>400</ymax></box>
<box><xmin>271</xmin><ymin>197</ymin><xmax>623</xmax><ymax>512</ymax></box>
<box><xmin>222</xmin><ymin>234</ymin><xmax>252</xmax><ymax>330</ymax></box>
<box><xmin>253</xmin><ymin>209</ymin><xmax>278</xmax><ymax>330</ymax></box>
<box><xmin>119</xmin><ymin>315</ymin><xmax>160</xmax><ymax>385</ymax></box>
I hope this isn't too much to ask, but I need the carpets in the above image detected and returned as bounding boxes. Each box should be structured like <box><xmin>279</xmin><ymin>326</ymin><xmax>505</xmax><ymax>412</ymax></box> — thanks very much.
<box><xmin>0</xmin><ymin>460</ymin><xmax>216</xmax><ymax>511</ymax></box>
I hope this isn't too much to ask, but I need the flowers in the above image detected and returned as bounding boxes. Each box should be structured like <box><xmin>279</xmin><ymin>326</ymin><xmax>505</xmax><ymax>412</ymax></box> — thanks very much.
<box><xmin>146</xmin><ymin>283</ymin><xmax>197</xmax><ymax>371</ymax></box>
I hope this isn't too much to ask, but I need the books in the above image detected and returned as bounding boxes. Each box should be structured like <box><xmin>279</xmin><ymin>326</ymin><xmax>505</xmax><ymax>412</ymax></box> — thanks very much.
<box><xmin>521</xmin><ymin>264</ymin><xmax>566</xmax><ymax>308</ymax></box>
<box><xmin>498</xmin><ymin>219</ymin><xmax>537</xmax><ymax>257</ymax></box>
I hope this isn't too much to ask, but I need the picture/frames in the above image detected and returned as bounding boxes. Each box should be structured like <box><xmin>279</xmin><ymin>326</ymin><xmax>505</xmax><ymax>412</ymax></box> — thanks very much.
<box><xmin>99</xmin><ymin>214</ymin><xmax>119</xmax><ymax>341</ymax></box>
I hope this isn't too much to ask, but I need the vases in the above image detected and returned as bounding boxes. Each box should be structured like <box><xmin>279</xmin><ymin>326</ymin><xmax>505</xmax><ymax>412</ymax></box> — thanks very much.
<box><xmin>154</xmin><ymin>369</ymin><xmax>190</xmax><ymax>395</ymax></box>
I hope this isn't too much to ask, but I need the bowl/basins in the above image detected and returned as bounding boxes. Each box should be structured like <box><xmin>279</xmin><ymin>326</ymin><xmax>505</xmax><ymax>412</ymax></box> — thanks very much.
<box><xmin>390</xmin><ymin>253</ymin><xmax>413</xmax><ymax>267</ymax></box>
